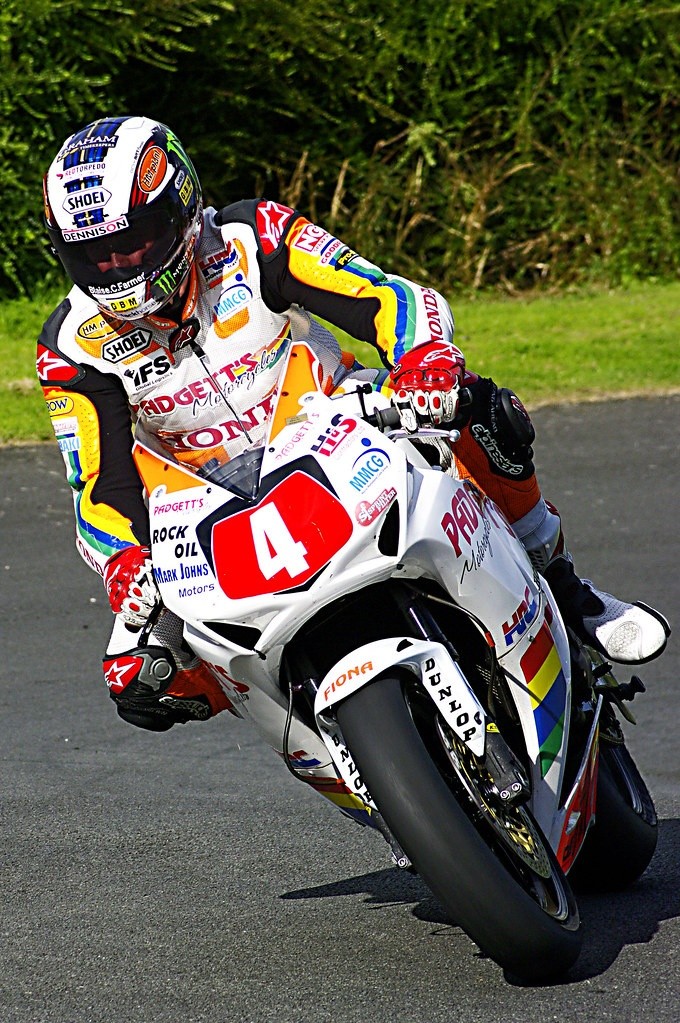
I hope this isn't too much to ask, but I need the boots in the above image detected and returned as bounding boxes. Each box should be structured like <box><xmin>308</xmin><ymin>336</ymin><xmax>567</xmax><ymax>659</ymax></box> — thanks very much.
<box><xmin>521</xmin><ymin>498</ymin><xmax>672</xmax><ymax>665</ymax></box>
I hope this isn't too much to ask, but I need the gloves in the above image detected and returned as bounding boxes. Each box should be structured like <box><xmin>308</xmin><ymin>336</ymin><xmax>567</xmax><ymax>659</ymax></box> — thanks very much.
<box><xmin>389</xmin><ymin>341</ymin><xmax>467</xmax><ymax>435</ymax></box>
<box><xmin>102</xmin><ymin>544</ymin><xmax>165</xmax><ymax>630</ymax></box>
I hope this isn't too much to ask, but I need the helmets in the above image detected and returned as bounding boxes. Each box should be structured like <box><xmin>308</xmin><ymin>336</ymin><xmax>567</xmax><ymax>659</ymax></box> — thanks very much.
<box><xmin>42</xmin><ymin>114</ymin><xmax>204</xmax><ymax>321</ymax></box>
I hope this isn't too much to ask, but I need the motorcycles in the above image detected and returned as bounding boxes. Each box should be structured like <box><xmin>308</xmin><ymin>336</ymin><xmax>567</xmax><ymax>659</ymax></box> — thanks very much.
<box><xmin>132</xmin><ymin>388</ymin><xmax>667</xmax><ymax>984</ymax></box>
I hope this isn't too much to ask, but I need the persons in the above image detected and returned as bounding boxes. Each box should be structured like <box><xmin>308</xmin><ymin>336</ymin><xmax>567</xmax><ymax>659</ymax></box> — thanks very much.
<box><xmin>33</xmin><ymin>113</ymin><xmax>673</xmax><ymax>731</ymax></box>
